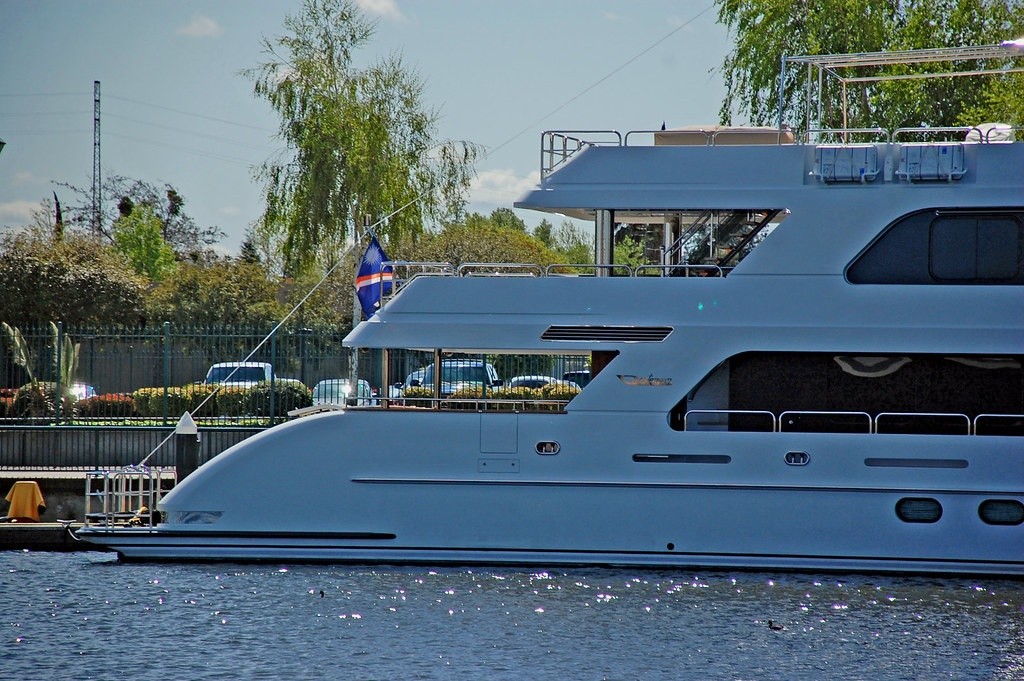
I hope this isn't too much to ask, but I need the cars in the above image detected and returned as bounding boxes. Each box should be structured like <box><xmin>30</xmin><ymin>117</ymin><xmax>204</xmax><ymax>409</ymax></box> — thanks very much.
<box><xmin>379</xmin><ymin>369</ymin><xmax>424</xmax><ymax>399</ymax></box>
<box><xmin>509</xmin><ymin>370</ymin><xmax>589</xmax><ymax>393</ymax></box>
<box><xmin>0</xmin><ymin>380</ymin><xmax>95</xmax><ymax>408</ymax></box>
<box><xmin>312</xmin><ymin>379</ymin><xmax>377</xmax><ymax>407</ymax></box>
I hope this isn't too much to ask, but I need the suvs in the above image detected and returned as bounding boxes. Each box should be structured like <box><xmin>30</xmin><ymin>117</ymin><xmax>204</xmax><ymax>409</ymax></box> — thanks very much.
<box><xmin>411</xmin><ymin>360</ymin><xmax>504</xmax><ymax>392</ymax></box>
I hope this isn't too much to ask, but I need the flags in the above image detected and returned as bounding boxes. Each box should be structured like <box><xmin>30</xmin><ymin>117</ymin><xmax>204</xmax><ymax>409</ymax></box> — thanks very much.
<box><xmin>353</xmin><ymin>237</ymin><xmax>399</xmax><ymax>320</ymax></box>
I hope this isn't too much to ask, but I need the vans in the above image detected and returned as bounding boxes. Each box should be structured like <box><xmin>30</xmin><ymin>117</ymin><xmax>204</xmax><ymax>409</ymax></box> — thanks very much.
<box><xmin>196</xmin><ymin>362</ymin><xmax>276</xmax><ymax>387</ymax></box>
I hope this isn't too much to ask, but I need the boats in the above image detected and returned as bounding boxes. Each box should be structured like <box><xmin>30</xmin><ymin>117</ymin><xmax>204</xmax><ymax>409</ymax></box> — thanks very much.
<box><xmin>61</xmin><ymin>39</ymin><xmax>1023</xmax><ymax>579</ymax></box>
